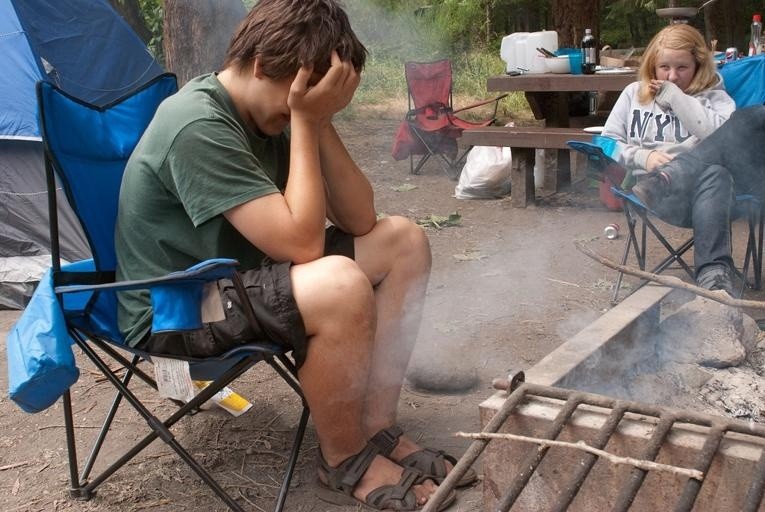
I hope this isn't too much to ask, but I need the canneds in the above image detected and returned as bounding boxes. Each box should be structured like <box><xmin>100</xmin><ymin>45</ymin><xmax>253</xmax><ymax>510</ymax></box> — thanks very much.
<box><xmin>604</xmin><ymin>222</ymin><xmax>620</xmax><ymax>239</ymax></box>
<box><xmin>588</xmin><ymin>92</ymin><xmax>599</xmax><ymax>115</ymax></box>
<box><xmin>726</xmin><ymin>47</ymin><xmax>737</xmax><ymax>63</ymax></box>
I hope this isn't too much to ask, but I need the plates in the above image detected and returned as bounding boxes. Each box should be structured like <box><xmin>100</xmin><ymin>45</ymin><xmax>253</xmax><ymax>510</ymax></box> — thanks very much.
<box><xmin>582</xmin><ymin>127</ymin><xmax>605</xmax><ymax>134</ymax></box>
<box><xmin>595</xmin><ymin>70</ymin><xmax>636</xmax><ymax>74</ymax></box>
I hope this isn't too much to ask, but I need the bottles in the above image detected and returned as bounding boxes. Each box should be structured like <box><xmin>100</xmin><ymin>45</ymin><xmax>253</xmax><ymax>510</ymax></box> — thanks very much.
<box><xmin>582</xmin><ymin>29</ymin><xmax>596</xmax><ymax>74</ymax></box>
<box><xmin>749</xmin><ymin>15</ymin><xmax>762</xmax><ymax>57</ymax></box>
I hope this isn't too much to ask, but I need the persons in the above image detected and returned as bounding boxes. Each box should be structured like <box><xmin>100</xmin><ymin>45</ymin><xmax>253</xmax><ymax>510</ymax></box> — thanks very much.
<box><xmin>115</xmin><ymin>1</ymin><xmax>475</xmax><ymax>511</ymax></box>
<box><xmin>598</xmin><ymin>25</ymin><xmax>765</xmax><ymax>298</ymax></box>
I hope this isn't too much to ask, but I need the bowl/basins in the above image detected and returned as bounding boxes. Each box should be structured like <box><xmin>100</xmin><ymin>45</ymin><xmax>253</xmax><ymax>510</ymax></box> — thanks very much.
<box><xmin>545</xmin><ymin>56</ymin><xmax>570</xmax><ymax>73</ymax></box>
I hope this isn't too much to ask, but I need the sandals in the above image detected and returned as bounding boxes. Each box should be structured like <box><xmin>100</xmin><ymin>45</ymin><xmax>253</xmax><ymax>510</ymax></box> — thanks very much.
<box><xmin>313</xmin><ymin>443</ymin><xmax>455</xmax><ymax>512</ymax></box>
<box><xmin>370</xmin><ymin>426</ymin><xmax>476</xmax><ymax>487</ymax></box>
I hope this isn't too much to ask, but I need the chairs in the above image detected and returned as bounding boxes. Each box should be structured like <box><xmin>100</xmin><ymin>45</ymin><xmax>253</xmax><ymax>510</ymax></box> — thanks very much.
<box><xmin>391</xmin><ymin>58</ymin><xmax>507</xmax><ymax>179</ymax></box>
<box><xmin>566</xmin><ymin>54</ymin><xmax>765</xmax><ymax>306</ymax></box>
<box><xmin>5</xmin><ymin>72</ymin><xmax>311</xmax><ymax>512</ymax></box>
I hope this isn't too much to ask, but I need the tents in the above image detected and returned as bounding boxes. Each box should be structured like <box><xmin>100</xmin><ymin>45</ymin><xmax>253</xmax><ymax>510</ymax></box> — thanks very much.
<box><xmin>0</xmin><ymin>0</ymin><xmax>163</xmax><ymax>310</ymax></box>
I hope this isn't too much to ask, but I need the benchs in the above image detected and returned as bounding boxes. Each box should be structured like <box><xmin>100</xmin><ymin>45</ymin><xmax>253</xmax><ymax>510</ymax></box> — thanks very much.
<box><xmin>462</xmin><ymin>128</ymin><xmax>597</xmax><ymax>209</ymax></box>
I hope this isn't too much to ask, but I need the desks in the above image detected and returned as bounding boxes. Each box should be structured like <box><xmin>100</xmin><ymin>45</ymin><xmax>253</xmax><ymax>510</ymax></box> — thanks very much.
<box><xmin>487</xmin><ymin>74</ymin><xmax>645</xmax><ymax>192</ymax></box>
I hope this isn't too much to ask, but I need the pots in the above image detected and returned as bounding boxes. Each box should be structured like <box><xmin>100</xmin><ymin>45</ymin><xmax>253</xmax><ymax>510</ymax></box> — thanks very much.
<box><xmin>655</xmin><ymin>0</ymin><xmax>715</xmax><ymax>18</ymax></box>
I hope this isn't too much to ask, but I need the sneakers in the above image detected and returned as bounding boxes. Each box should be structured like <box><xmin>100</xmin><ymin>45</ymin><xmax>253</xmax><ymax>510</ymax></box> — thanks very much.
<box><xmin>697</xmin><ymin>269</ymin><xmax>733</xmax><ymax>294</ymax></box>
<box><xmin>632</xmin><ymin>172</ymin><xmax>668</xmax><ymax>209</ymax></box>
